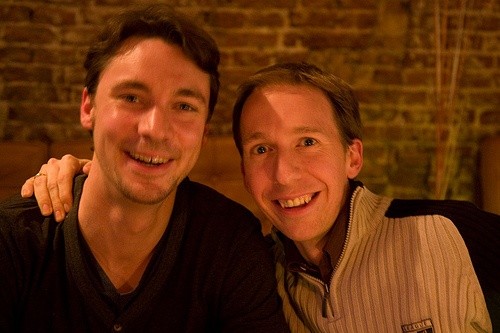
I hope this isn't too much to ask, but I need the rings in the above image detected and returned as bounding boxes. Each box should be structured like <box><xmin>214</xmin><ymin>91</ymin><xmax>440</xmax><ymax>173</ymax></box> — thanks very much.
<box><xmin>32</xmin><ymin>172</ymin><xmax>47</xmax><ymax>178</ymax></box>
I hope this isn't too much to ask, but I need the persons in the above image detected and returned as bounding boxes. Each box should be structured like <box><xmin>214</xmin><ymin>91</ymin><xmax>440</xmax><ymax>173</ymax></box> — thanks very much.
<box><xmin>21</xmin><ymin>63</ymin><xmax>500</xmax><ymax>333</ymax></box>
<box><xmin>0</xmin><ymin>6</ymin><xmax>290</xmax><ymax>333</ymax></box>
